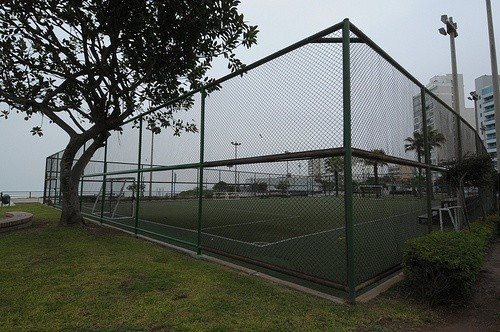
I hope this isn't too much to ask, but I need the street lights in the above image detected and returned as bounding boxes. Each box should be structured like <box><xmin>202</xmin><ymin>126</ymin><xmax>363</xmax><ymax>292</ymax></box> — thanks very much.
<box><xmin>468</xmin><ymin>91</ymin><xmax>479</xmax><ymax>158</ymax></box>
<box><xmin>285</xmin><ymin>150</ymin><xmax>290</xmax><ymax>176</ymax></box>
<box><xmin>231</xmin><ymin>142</ymin><xmax>241</xmax><ymax>191</ymax></box>
<box><xmin>440</xmin><ymin>15</ymin><xmax>463</xmax><ymax>189</ymax></box>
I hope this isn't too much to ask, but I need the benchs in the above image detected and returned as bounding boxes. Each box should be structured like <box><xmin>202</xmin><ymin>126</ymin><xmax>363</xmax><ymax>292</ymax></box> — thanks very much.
<box><xmin>419</xmin><ymin>193</ymin><xmax>479</xmax><ymax>226</ymax></box>
<box><xmin>1</xmin><ymin>211</ymin><xmax>33</xmax><ymax>233</ymax></box>
